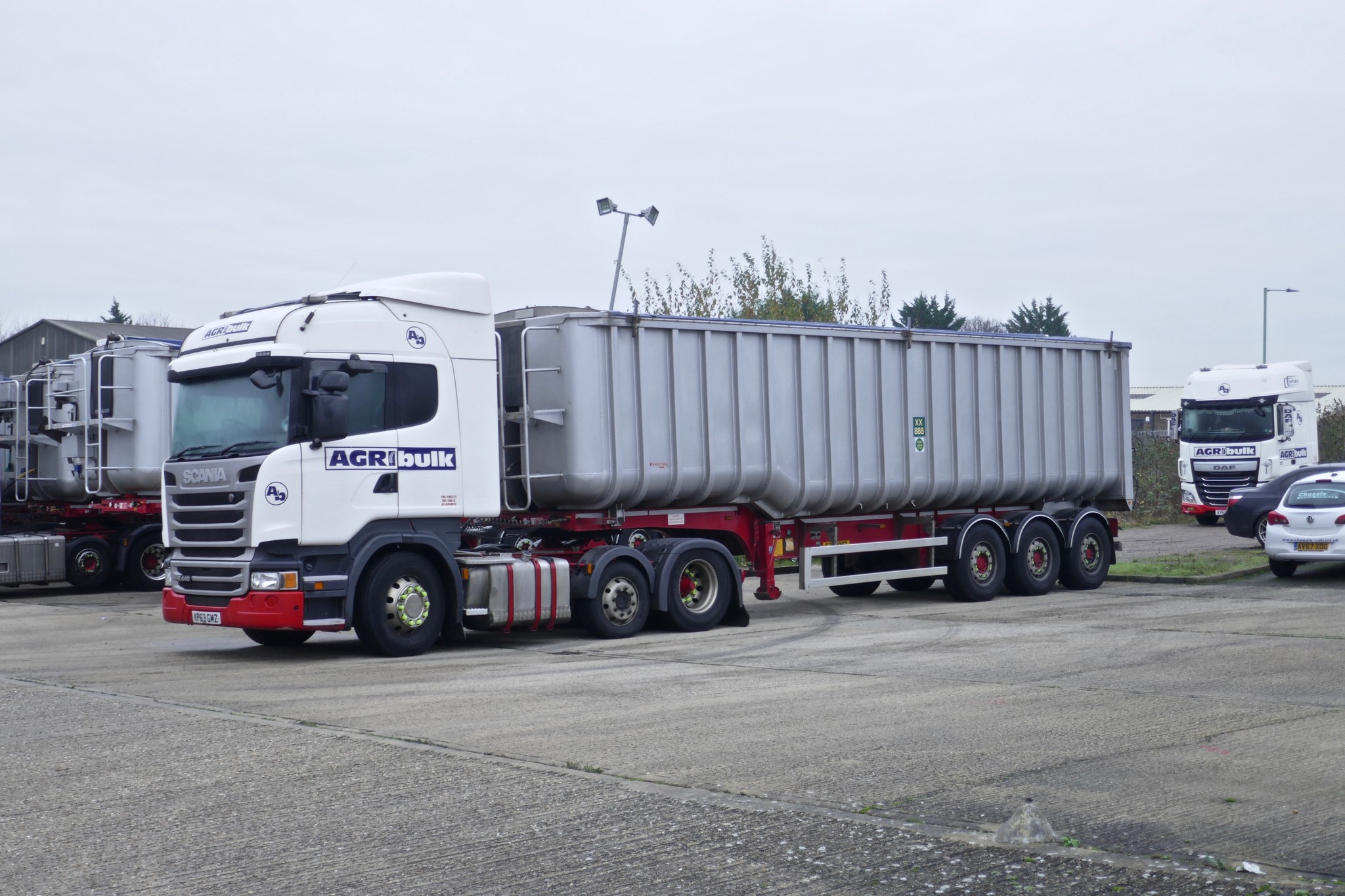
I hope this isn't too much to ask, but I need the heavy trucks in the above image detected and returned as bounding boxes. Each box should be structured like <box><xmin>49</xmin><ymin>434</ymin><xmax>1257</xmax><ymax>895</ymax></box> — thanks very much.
<box><xmin>1180</xmin><ymin>359</ymin><xmax>1319</xmax><ymax>527</ymax></box>
<box><xmin>164</xmin><ymin>273</ymin><xmax>1135</xmax><ymax>656</ymax></box>
<box><xmin>1</xmin><ymin>333</ymin><xmax>181</xmax><ymax>596</ymax></box>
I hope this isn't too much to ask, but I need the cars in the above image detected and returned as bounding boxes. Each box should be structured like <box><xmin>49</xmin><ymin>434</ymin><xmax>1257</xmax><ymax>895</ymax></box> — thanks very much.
<box><xmin>1223</xmin><ymin>462</ymin><xmax>1345</xmax><ymax>547</ymax></box>
<box><xmin>1264</xmin><ymin>471</ymin><xmax>1345</xmax><ymax>580</ymax></box>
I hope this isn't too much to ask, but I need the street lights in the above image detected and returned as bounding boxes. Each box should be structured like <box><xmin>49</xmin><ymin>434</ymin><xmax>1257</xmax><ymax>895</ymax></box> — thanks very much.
<box><xmin>596</xmin><ymin>197</ymin><xmax>660</xmax><ymax>311</ymax></box>
<box><xmin>1262</xmin><ymin>287</ymin><xmax>1300</xmax><ymax>364</ymax></box>
